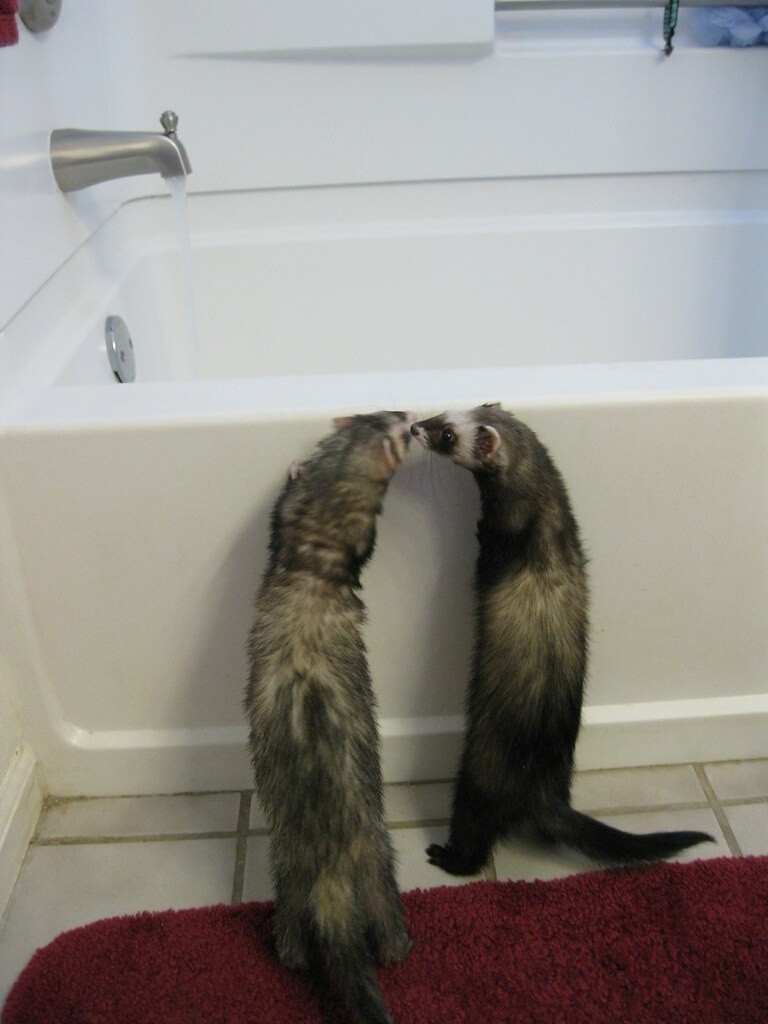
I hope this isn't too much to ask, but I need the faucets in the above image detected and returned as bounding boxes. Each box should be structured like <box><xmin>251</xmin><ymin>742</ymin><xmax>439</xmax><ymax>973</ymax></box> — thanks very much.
<box><xmin>50</xmin><ymin>108</ymin><xmax>193</xmax><ymax>194</ymax></box>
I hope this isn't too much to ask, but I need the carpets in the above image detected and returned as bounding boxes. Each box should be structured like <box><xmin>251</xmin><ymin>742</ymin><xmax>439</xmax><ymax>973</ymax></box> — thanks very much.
<box><xmin>1</xmin><ymin>853</ymin><xmax>768</xmax><ymax>1024</ymax></box>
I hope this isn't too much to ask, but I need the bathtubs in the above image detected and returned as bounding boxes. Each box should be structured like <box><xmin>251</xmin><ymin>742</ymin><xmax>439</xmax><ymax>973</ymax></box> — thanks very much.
<box><xmin>0</xmin><ymin>166</ymin><xmax>765</xmax><ymax>799</ymax></box>
